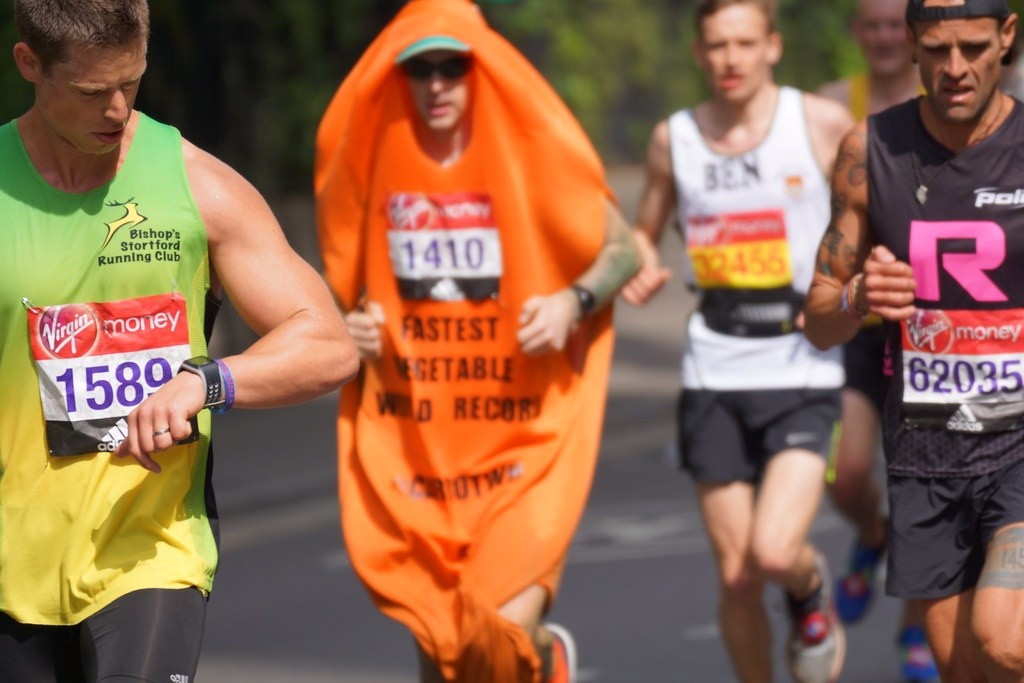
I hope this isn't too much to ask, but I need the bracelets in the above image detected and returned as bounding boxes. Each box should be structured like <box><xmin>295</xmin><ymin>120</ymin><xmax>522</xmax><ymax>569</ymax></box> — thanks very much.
<box><xmin>841</xmin><ymin>274</ymin><xmax>868</xmax><ymax>320</ymax></box>
<box><xmin>570</xmin><ymin>284</ymin><xmax>595</xmax><ymax>320</ymax></box>
<box><xmin>209</xmin><ymin>358</ymin><xmax>234</xmax><ymax>414</ymax></box>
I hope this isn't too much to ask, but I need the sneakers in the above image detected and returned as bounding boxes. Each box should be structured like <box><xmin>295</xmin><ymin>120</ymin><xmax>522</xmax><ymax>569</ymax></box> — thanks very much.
<box><xmin>788</xmin><ymin>554</ymin><xmax>845</xmax><ymax>682</ymax></box>
<box><xmin>834</xmin><ymin>560</ymin><xmax>877</xmax><ymax>624</ymax></box>
<box><xmin>896</xmin><ymin>628</ymin><xmax>935</xmax><ymax>682</ymax></box>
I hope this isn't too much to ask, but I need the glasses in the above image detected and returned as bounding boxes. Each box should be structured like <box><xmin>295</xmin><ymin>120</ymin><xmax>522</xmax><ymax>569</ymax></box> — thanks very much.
<box><xmin>400</xmin><ymin>55</ymin><xmax>468</xmax><ymax>78</ymax></box>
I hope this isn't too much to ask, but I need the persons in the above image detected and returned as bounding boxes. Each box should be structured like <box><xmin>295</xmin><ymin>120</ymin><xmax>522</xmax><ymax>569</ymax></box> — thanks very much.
<box><xmin>318</xmin><ymin>0</ymin><xmax>642</xmax><ymax>683</ymax></box>
<box><xmin>0</xmin><ymin>0</ymin><xmax>359</xmax><ymax>682</ymax></box>
<box><xmin>617</xmin><ymin>0</ymin><xmax>1024</xmax><ymax>683</ymax></box>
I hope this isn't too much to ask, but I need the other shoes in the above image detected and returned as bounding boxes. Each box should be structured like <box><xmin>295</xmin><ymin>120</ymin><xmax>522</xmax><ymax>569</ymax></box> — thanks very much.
<box><xmin>545</xmin><ymin>623</ymin><xmax>575</xmax><ymax>681</ymax></box>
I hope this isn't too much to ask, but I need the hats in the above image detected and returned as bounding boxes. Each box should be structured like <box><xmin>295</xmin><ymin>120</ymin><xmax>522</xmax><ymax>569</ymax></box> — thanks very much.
<box><xmin>906</xmin><ymin>0</ymin><xmax>1018</xmax><ymax>64</ymax></box>
<box><xmin>397</xmin><ymin>35</ymin><xmax>471</xmax><ymax>65</ymax></box>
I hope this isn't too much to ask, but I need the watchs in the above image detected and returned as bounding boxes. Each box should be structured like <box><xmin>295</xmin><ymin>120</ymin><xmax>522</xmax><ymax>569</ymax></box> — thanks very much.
<box><xmin>178</xmin><ymin>356</ymin><xmax>226</xmax><ymax>409</ymax></box>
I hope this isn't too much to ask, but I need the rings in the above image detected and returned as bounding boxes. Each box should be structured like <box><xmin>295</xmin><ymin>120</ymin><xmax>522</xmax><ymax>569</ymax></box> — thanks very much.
<box><xmin>154</xmin><ymin>429</ymin><xmax>169</xmax><ymax>435</ymax></box>
